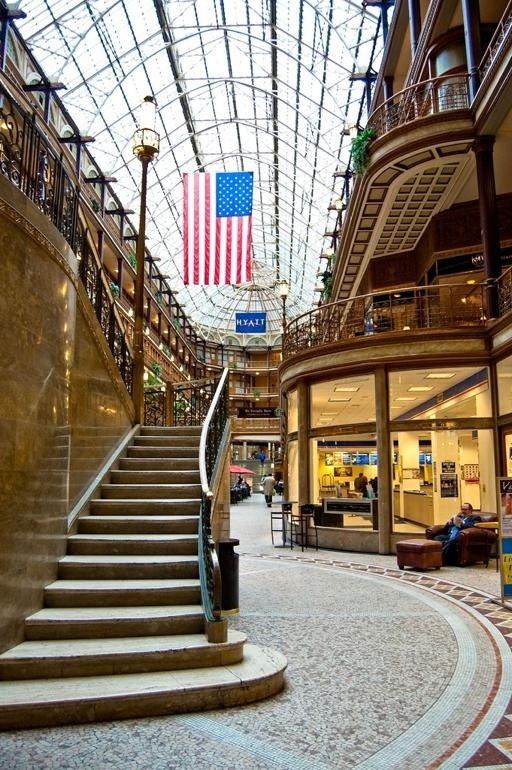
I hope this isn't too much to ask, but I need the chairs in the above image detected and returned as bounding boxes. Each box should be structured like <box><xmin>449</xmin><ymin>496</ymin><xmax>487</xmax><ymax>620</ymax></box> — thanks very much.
<box><xmin>269</xmin><ymin>500</ymin><xmax>298</xmax><ymax>548</ymax></box>
<box><xmin>230</xmin><ymin>482</ymin><xmax>251</xmax><ymax>505</ymax></box>
<box><xmin>289</xmin><ymin>502</ymin><xmax>320</xmax><ymax>552</ymax></box>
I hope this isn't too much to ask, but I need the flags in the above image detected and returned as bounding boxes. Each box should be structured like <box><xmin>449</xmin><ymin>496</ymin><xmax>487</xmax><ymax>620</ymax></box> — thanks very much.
<box><xmin>180</xmin><ymin>170</ymin><xmax>255</xmax><ymax>288</ymax></box>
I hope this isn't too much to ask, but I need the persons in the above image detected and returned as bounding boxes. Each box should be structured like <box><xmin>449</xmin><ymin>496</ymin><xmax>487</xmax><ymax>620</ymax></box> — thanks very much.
<box><xmin>354</xmin><ymin>473</ymin><xmax>367</xmax><ymax>497</ymax></box>
<box><xmin>432</xmin><ymin>502</ymin><xmax>482</xmax><ymax>567</ymax></box>
<box><xmin>235</xmin><ymin>473</ymin><xmax>275</xmax><ymax>508</ymax></box>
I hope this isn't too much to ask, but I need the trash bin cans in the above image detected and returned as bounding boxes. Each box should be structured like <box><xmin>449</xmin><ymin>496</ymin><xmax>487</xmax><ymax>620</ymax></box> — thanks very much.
<box><xmin>216</xmin><ymin>538</ymin><xmax>240</xmax><ymax>615</ymax></box>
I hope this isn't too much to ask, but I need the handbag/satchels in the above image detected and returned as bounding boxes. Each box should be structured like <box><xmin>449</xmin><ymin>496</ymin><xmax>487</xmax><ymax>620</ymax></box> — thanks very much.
<box><xmin>273</xmin><ymin>489</ymin><xmax>276</xmax><ymax>496</ymax></box>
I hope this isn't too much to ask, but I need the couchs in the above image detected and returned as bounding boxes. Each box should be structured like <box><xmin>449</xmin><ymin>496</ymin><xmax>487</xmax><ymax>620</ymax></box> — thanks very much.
<box><xmin>394</xmin><ymin>538</ymin><xmax>444</xmax><ymax>574</ymax></box>
<box><xmin>426</xmin><ymin>510</ymin><xmax>499</xmax><ymax>570</ymax></box>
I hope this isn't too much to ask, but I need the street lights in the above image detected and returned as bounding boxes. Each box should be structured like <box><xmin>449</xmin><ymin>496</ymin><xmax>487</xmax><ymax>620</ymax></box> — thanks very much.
<box><xmin>335</xmin><ymin>198</ymin><xmax>344</xmax><ymax>227</ymax></box>
<box><xmin>280</xmin><ymin>279</ymin><xmax>289</xmax><ymax>332</ymax></box>
<box><xmin>348</xmin><ymin>125</ymin><xmax>358</xmax><ymax>142</ymax></box>
<box><xmin>130</xmin><ymin>97</ymin><xmax>161</xmax><ymax>425</ymax></box>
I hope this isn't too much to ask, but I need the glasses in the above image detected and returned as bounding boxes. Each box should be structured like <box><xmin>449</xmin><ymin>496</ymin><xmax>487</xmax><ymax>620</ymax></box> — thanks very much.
<box><xmin>461</xmin><ymin>508</ymin><xmax>471</xmax><ymax>511</ymax></box>
